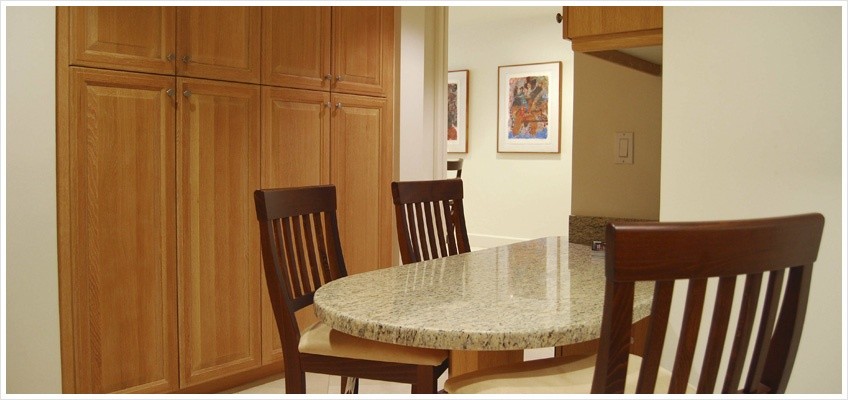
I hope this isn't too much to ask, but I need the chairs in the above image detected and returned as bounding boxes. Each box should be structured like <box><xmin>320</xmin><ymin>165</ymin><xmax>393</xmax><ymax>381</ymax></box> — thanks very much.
<box><xmin>386</xmin><ymin>181</ymin><xmax>471</xmax><ymax>263</ymax></box>
<box><xmin>252</xmin><ymin>183</ymin><xmax>452</xmax><ymax>394</ymax></box>
<box><xmin>427</xmin><ymin>211</ymin><xmax>824</xmax><ymax>394</ymax></box>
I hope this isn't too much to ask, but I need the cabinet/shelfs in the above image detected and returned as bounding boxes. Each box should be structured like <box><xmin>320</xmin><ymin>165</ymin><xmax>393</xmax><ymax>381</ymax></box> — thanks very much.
<box><xmin>57</xmin><ymin>6</ymin><xmax>402</xmax><ymax>394</ymax></box>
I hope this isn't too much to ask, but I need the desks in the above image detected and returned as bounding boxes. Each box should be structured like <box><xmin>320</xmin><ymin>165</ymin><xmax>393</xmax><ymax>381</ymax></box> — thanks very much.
<box><xmin>311</xmin><ymin>234</ymin><xmax>658</xmax><ymax>377</ymax></box>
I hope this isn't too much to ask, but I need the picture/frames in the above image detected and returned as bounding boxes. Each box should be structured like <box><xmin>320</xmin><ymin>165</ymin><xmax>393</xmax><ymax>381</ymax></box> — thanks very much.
<box><xmin>447</xmin><ymin>70</ymin><xmax>470</xmax><ymax>154</ymax></box>
<box><xmin>497</xmin><ymin>60</ymin><xmax>562</xmax><ymax>154</ymax></box>
